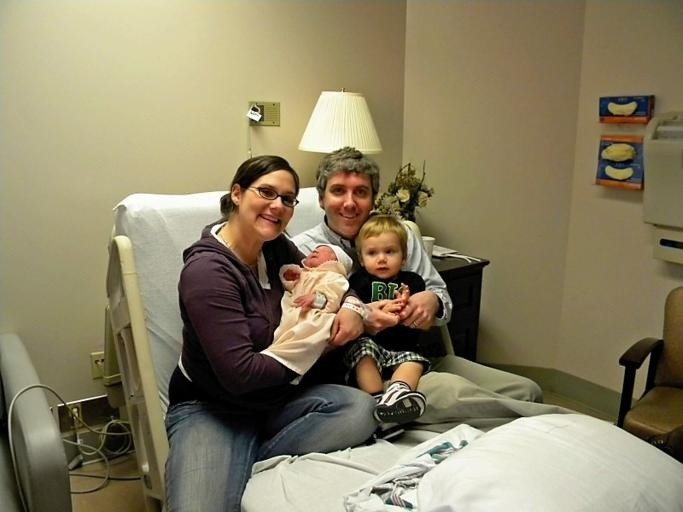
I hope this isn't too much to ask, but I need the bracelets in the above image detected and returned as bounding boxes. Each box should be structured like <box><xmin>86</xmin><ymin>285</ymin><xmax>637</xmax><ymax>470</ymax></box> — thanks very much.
<box><xmin>339</xmin><ymin>302</ymin><xmax>367</xmax><ymax>321</ymax></box>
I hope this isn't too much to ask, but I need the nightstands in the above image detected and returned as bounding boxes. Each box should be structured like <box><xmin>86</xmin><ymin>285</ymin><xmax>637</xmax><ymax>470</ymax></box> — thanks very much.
<box><xmin>429</xmin><ymin>252</ymin><xmax>489</xmax><ymax>363</ymax></box>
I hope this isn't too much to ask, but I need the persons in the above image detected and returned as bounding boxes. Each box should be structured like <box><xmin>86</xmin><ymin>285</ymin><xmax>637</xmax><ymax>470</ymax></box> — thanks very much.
<box><xmin>332</xmin><ymin>211</ymin><xmax>434</xmax><ymax>445</ymax></box>
<box><xmin>280</xmin><ymin>145</ymin><xmax>582</xmax><ymax>437</ymax></box>
<box><xmin>162</xmin><ymin>153</ymin><xmax>380</xmax><ymax>511</ymax></box>
<box><xmin>254</xmin><ymin>242</ymin><xmax>353</xmax><ymax>383</ymax></box>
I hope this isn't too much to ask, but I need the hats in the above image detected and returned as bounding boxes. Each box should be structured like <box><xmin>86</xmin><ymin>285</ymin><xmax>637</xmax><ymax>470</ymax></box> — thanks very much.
<box><xmin>314</xmin><ymin>244</ymin><xmax>353</xmax><ymax>276</ymax></box>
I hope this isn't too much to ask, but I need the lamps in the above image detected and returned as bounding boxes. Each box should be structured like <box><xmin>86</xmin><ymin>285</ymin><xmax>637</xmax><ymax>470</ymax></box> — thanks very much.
<box><xmin>297</xmin><ymin>87</ymin><xmax>384</xmax><ymax>158</ymax></box>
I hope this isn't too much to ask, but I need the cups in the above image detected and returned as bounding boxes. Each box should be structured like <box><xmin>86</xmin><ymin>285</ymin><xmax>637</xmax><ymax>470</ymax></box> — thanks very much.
<box><xmin>422</xmin><ymin>235</ymin><xmax>436</xmax><ymax>265</ymax></box>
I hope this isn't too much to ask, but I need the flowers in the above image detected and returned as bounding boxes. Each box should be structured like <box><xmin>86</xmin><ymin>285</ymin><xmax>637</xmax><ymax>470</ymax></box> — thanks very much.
<box><xmin>375</xmin><ymin>159</ymin><xmax>436</xmax><ymax>223</ymax></box>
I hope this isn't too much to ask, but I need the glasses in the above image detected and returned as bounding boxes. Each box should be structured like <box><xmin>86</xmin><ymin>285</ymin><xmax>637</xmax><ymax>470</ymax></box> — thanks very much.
<box><xmin>250</xmin><ymin>186</ymin><xmax>299</xmax><ymax>208</ymax></box>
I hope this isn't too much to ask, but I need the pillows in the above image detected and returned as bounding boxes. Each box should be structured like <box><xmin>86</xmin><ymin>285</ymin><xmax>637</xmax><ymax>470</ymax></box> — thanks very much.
<box><xmin>406</xmin><ymin>410</ymin><xmax>682</xmax><ymax>509</ymax></box>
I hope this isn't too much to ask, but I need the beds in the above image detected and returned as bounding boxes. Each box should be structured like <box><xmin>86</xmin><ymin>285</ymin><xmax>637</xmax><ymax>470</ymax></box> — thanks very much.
<box><xmin>99</xmin><ymin>187</ymin><xmax>682</xmax><ymax>510</ymax></box>
<box><xmin>0</xmin><ymin>331</ymin><xmax>73</xmax><ymax>512</ymax></box>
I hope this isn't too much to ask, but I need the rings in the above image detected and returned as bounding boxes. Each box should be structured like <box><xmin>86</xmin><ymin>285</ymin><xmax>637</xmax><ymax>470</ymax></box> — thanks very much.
<box><xmin>412</xmin><ymin>320</ymin><xmax>418</xmax><ymax>328</ymax></box>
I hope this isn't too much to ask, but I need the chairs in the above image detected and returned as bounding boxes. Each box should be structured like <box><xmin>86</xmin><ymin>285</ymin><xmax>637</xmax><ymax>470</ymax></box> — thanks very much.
<box><xmin>617</xmin><ymin>281</ymin><xmax>683</xmax><ymax>462</ymax></box>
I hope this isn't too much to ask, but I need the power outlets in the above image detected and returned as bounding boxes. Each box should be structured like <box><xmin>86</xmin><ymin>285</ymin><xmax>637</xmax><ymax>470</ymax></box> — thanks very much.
<box><xmin>57</xmin><ymin>402</ymin><xmax>84</xmax><ymax>434</ymax></box>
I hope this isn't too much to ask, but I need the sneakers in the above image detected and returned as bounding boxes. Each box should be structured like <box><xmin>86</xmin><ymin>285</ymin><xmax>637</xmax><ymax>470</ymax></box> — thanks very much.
<box><xmin>370</xmin><ymin>380</ymin><xmax>427</xmax><ymax>443</ymax></box>
